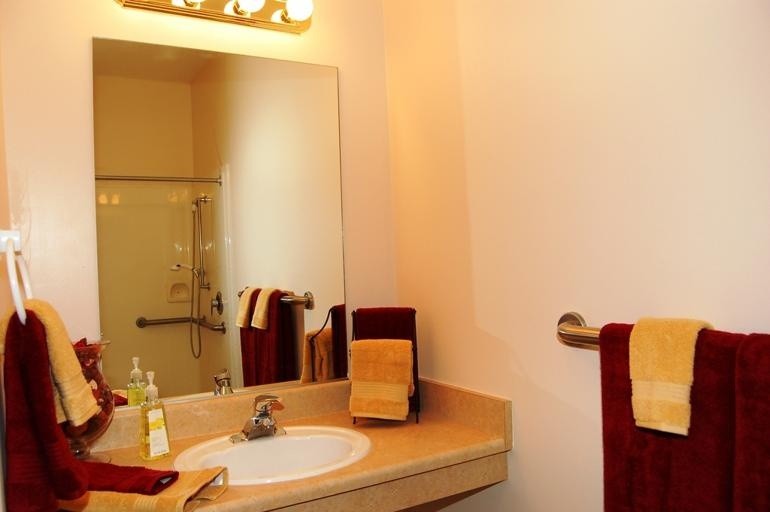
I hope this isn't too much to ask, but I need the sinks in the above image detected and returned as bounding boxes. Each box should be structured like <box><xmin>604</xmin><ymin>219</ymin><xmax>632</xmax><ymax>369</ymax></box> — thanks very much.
<box><xmin>191</xmin><ymin>436</ymin><xmax>351</xmax><ymax>482</ymax></box>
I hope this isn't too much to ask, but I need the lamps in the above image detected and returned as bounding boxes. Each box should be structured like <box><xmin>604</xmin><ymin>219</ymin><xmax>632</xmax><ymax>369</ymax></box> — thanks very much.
<box><xmin>271</xmin><ymin>1</ymin><xmax>314</xmax><ymax>31</ymax></box>
<box><xmin>173</xmin><ymin>2</ymin><xmax>207</xmax><ymax>16</ymax></box>
<box><xmin>224</xmin><ymin>0</ymin><xmax>266</xmax><ymax>24</ymax></box>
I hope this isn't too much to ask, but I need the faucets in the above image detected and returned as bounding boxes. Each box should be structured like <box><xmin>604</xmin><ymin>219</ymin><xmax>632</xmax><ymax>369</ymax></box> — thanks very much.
<box><xmin>212</xmin><ymin>368</ymin><xmax>233</xmax><ymax>396</ymax></box>
<box><xmin>228</xmin><ymin>395</ymin><xmax>288</xmax><ymax>443</ymax></box>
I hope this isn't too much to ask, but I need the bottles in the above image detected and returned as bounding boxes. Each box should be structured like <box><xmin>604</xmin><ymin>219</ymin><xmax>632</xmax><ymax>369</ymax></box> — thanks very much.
<box><xmin>57</xmin><ymin>341</ymin><xmax>117</xmax><ymax>471</ymax></box>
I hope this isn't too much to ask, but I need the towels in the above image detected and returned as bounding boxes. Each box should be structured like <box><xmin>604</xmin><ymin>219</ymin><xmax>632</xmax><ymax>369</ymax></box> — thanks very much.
<box><xmin>326</xmin><ymin>306</ymin><xmax>348</xmax><ymax>379</ymax></box>
<box><xmin>4</xmin><ymin>299</ymin><xmax>229</xmax><ymax>512</ymax></box>
<box><xmin>232</xmin><ymin>288</ymin><xmax>249</xmax><ymax>330</ymax></box>
<box><xmin>253</xmin><ymin>285</ymin><xmax>281</xmax><ymax>329</ymax></box>
<box><xmin>300</xmin><ymin>329</ymin><xmax>329</xmax><ymax>380</ymax></box>
<box><xmin>598</xmin><ymin>317</ymin><xmax>770</xmax><ymax>512</ymax></box>
<box><xmin>348</xmin><ymin>340</ymin><xmax>417</xmax><ymax>418</ymax></box>
<box><xmin>628</xmin><ymin>313</ymin><xmax>698</xmax><ymax>438</ymax></box>
<box><xmin>235</xmin><ymin>289</ymin><xmax>289</xmax><ymax>386</ymax></box>
<box><xmin>351</xmin><ymin>306</ymin><xmax>419</xmax><ymax>339</ymax></box>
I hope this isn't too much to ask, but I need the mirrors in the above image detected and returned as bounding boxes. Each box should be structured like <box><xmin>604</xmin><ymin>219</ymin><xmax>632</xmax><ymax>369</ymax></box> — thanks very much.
<box><xmin>90</xmin><ymin>37</ymin><xmax>350</xmax><ymax>407</ymax></box>
